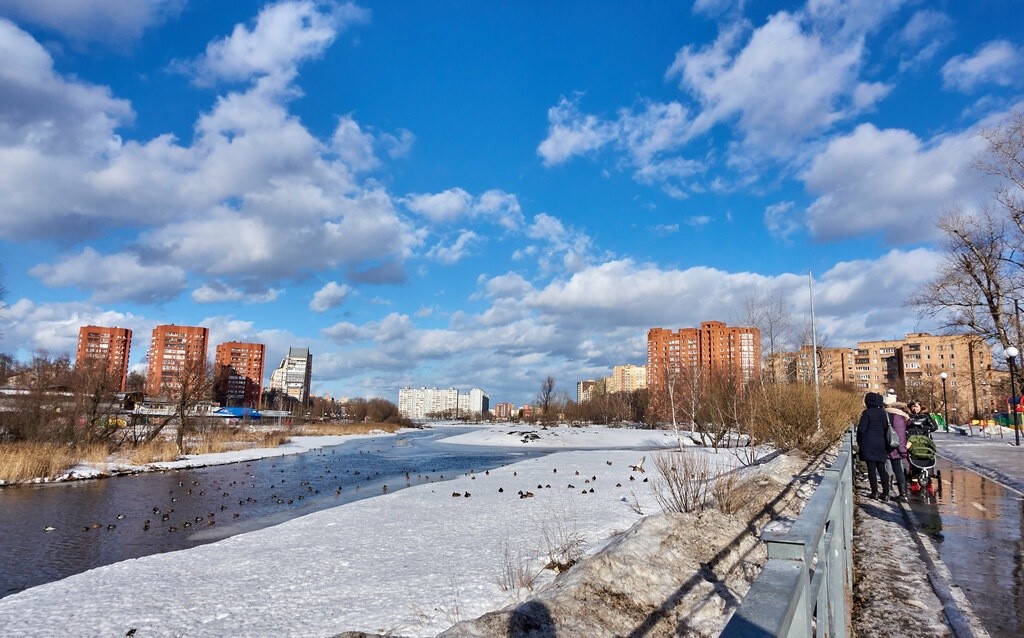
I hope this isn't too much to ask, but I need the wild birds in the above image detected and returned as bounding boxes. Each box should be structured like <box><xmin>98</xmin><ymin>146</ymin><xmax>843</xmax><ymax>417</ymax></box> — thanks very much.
<box><xmin>627</xmin><ymin>456</ymin><xmax>646</xmax><ymax>475</ymax></box>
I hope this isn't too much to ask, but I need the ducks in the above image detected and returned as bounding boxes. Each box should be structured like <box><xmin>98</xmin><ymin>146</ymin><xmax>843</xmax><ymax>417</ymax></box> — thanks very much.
<box><xmin>41</xmin><ymin>439</ymin><xmax>651</xmax><ymax>540</ymax></box>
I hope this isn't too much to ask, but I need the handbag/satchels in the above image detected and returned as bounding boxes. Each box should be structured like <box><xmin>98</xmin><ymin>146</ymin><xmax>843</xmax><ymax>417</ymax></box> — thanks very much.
<box><xmin>886</xmin><ymin>411</ymin><xmax>900</xmax><ymax>450</ymax></box>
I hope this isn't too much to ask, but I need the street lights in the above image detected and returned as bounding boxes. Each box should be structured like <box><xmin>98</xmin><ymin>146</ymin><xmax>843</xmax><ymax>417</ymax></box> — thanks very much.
<box><xmin>940</xmin><ymin>372</ymin><xmax>952</xmax><ymax>432</ymax></box>
<box><xmin>1003</xmin><ymin>346</ymin><xmax>1021</xmax><ymax>447</ymax></box>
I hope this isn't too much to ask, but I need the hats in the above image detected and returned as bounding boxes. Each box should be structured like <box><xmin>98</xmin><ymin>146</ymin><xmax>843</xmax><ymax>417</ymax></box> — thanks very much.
<box><xmin>865</xmin><ymin>392</ymin><xmax>883</xmax><ymax>407</ymax></box>
<box><xmin>882</xmin><ymin>388</ymin><xmax>897</xmax><ymax>405</ymax></box>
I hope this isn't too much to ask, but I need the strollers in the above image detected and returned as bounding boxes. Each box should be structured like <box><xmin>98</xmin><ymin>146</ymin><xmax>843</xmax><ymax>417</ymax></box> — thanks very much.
<box><xmin>906</xmin><ymin>424</ymin><xmax>944</xmax><ymax>496</ymax></box>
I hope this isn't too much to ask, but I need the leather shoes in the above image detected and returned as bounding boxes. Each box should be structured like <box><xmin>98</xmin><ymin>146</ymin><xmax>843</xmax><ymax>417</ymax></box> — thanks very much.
<box><xmin>867</xmin><ymin>493</ymin><xmax>878</xmax><ymax>500</ymax></box>
<box><xmin>878</xmin><ymin>494</ymin><xmax>888</xmax><ymax>502</ymax></box>
<box><xmin>893</xmin><ymin>494</ymin><xmax>909</xmax><ymax>502</ymax></box>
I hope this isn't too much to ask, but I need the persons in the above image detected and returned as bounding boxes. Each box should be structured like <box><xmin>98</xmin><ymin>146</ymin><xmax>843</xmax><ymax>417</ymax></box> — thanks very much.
<box><xmin>883</xmin><ymin>388</ymin><xmax>910</xmax><ymax>502</ymax></box>
<box><xmin>905</xmin><ymin>399</ymin><xmax>940</xmax><ymax>497</ymax></box>
<box><xmin>857</xmin><ymin>391</ymin><xmax>892</xmax><ymax>501</ymax></box>
